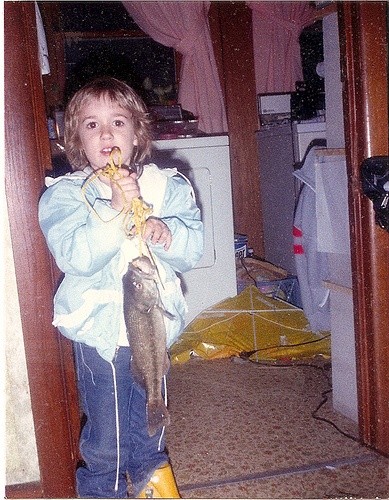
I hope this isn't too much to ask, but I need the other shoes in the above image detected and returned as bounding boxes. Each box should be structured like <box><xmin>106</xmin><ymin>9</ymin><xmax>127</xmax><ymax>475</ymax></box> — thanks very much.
<box><xmin>134</xmin><ymin>460</ymin><xmax>181</xmax><ymax>498</ymax></box>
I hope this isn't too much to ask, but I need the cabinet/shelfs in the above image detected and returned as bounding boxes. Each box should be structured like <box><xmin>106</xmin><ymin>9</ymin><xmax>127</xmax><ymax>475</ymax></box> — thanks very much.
<box><xmin>146</xmin><ymin>136</ymin><xmax>237</xmax><ymax>328</ymax></box>
<box><xmin>254</xmin><ymin>123</ymin><xmax>326</xmax><ymax>277</ymax></box>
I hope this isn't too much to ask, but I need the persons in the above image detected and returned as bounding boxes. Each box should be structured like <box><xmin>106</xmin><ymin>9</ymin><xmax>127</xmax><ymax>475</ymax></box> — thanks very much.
<box><xmin>38</xmin><ymin>77</ymin><xmax>204</xmax><ymax>499</ymax></box>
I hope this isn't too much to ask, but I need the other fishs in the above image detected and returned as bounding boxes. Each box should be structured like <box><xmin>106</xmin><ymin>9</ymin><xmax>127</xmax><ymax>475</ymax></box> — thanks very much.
<box><xmin>121</xmin><ymin>254</ymin><xmax>172</xmax><ymax>437</ymax></box>
